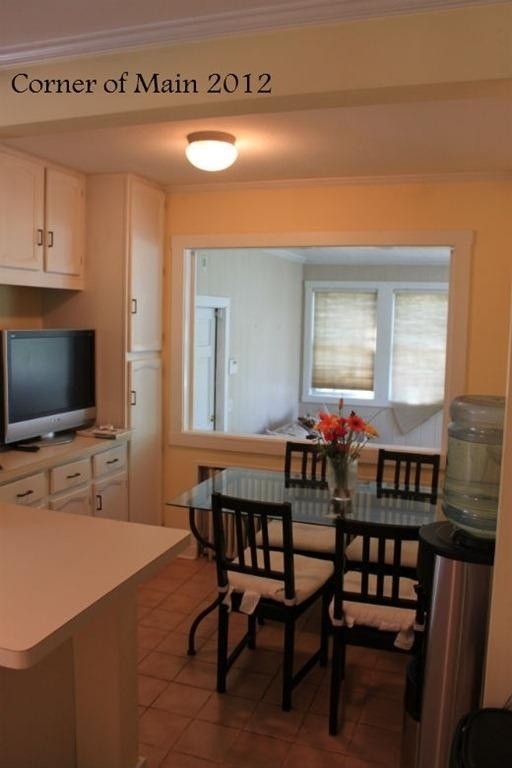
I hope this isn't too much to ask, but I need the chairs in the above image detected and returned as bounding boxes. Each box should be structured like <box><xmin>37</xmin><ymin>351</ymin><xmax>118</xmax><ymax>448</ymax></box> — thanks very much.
<box><xmin>165</xmin><ymin>441</ymin><xmax>449</xmax><ymax>735</ymax></box>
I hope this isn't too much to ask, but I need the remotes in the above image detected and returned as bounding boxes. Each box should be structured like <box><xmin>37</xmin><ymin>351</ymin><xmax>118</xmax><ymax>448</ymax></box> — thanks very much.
<box><xmin>13</xmin><ymin>445</ymin><xmax>40</xmax><ymax>451</ymax></box>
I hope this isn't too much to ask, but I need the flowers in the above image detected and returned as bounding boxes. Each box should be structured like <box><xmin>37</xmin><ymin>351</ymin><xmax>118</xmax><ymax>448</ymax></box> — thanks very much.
<box><xmin>312</xmin><ymin>398</ymin><xmax>379</xmax><ymax>513</ymax></box>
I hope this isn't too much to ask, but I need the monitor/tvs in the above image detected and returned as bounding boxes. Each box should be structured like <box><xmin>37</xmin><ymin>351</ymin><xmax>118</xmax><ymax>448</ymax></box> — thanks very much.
<box><xmin>2</xmin><ymin>328</ymin><xmax>97</xmax><ymax>447</ymax></box>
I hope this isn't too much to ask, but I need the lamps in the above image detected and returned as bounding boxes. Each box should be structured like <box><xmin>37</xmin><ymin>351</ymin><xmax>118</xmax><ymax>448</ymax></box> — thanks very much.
<box><xmin>185</xmin><ymin>131</ymin><xmax>238</xmax><ymax>172</ymax></box>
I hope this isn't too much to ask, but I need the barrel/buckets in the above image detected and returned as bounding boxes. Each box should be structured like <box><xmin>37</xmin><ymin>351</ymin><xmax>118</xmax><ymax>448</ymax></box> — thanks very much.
<box><xmin>439</xmin><ymin>394</ymin><xmax>505</xmax><ymax>540</ymax></box>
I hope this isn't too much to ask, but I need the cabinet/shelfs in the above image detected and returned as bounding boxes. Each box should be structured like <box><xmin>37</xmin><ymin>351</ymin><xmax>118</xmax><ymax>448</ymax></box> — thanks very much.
<box><xmin>127</xmin><ymin>359</ymin><xmax>164</xmax><ymax>529</ymax></box>
<box><xmin>126</xmin><ymin>173</ymin><xmax>165</xmax><ymax>356</ymax></box>
<box><xmin>0</xmin><ymin>140</ymin><xmax>87</xmax><ymax>294</ymax></box>
<box><xmin>0</xmin><ymin>440</ymin><xmax>129</xmax><ymax>522</ymax></box>
